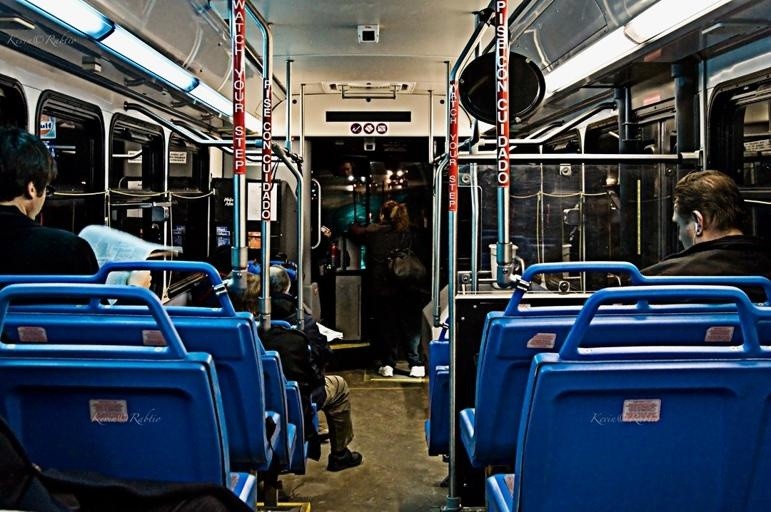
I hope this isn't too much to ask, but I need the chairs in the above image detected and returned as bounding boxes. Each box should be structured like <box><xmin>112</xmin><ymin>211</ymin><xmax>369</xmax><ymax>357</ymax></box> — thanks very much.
<box><xmin>425</xmin><ymin>262</ymin><xmax>771</xmax><ymax>512</ymax></box>
<box><xmin>377</xmin><ymin>365</ymin><xmax>394</xmax><ymax>377</ymax></box>
<box><xmin>0</xmin><ymin>258</ymin><xmax>317</xmax><ymax>512</ymax></box>
<box><xmin>409</xmin><ymin>365</ymin><xmax>426</xmax><ymax>376</ymax></box>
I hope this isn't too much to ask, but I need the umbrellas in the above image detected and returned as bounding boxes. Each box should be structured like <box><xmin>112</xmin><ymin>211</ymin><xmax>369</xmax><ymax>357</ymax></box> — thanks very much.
<box><xmin>327</xmin><ymin>448</ymin><xmax>362</xmax><ymax>472</ymax></box>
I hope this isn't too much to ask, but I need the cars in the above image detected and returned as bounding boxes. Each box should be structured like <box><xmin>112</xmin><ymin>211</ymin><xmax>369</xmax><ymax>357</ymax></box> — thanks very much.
<box><xmin>489</xmin><ymin>244</ymin><xmax>519</xmax><ymax>290</ymax></box>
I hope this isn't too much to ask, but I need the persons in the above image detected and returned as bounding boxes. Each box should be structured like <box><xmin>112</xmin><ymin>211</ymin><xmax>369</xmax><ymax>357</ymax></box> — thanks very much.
<box><xmin>622</xmin><ymin>169</ymin><xmax>770</xmax><ymax>308</ymax></box>
<box><xmin>192</xmin><ymin>249</ymin><xmax>364</xmax><ymax>473</ymax></box>
<box><xmin>335</xmin><ymin>160</ymin><xmax>354</xmax><ymax>178</ymax></box>
<box><xmin>352</xmin><ymin>199</ymin><xmax>430</xmax><ymax>379</ymax></box>
<box><xmin>0</xmin><ymin>127</ymin><xmax>154</xmax><ymax>308</ymax></box>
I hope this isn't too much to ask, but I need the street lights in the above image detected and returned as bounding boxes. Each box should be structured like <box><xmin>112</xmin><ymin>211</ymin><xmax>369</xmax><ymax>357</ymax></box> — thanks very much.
<box><xmin>388</xmin><ymin>247</ymin><xmax>426</xmax><ymax>279</ymax></box>
